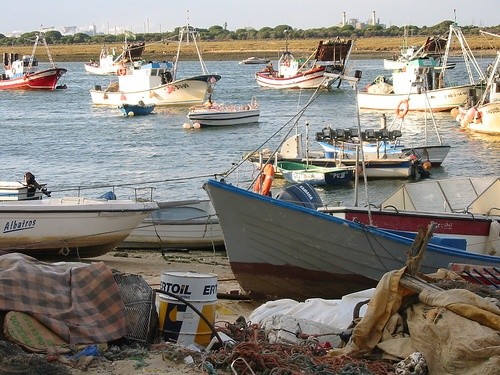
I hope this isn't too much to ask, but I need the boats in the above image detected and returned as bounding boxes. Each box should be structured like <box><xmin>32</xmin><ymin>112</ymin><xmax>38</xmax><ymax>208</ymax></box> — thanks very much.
<box><xmin>271</xmin><ymin>161</ymin><xmax>363</xmax><ymax>189</ymax></box>
<box><xmin>356</xmin><ymin>9</ymin><xmax>478</xmax><ymax>112</ymax></box>
<box><xmin>0</xmin><ymin>177</ymin><xmax>159</xmax><ymax>257</ymax></box>
<box><xmin>203</xmin><ymin>67</ymin><xmax>500</xmax><ymax>304</ymax></box>
<box><xmin>378</xmin><ymin>174</ymin><xmax>500</xmax><ymax>218</ymax></box>
<box><xmin>455</xmin><ymin>29</ymin><xmax>500</xmax><ymax>136</ymax></box>
<box><xmin>247</xmin><ymin>122</ymin><xmax>422</xmax><ymax>178</ymax></box>
<box><xmin>242</xmin><ymin>56</ymin><xmax>271</xmax><ymax>65</ymax></box>
<box><xmin>84</xmin><ymin>30</ymin><xmax>174</xmax><ymax>76</ymax></box>
<box><xmin>118</xmin><ymin>102</ymin><xmax>156</xmax><ymax>116</ymax></box>
<box><xmin>255</xmin><ymin>30</ymin><xmax>353</xmax><ymax>90</ymax></box>
<box><xmin>187</xmin><ymin>94</ymin><xmax>262</xmax><ymax>127</ymax></box>
<box><xmin>115</xmin><ymin>199</ymin><xmax>226</xmax><ymax>252</ymax></box>
<box><xmin>0</xmin><ymin>23</ymin><xmax>69</xmax><ymax>92</ymax></box>
<box><xmin>383</xmin><ymin>34</ymin><xmax>457</xmax><ymax>70</ymax></box>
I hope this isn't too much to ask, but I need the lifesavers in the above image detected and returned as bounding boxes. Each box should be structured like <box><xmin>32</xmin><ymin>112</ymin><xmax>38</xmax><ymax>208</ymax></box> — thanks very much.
<box><xmin>117</xmin><ymin>66</ymin><xmax>126</xmax><ymax>76</ymax></box>
<box><xmin>395</xmin><ymin>100</ymin><xmax>409</xmax><ymax>119</ymax></box>
<box><xmin>253</xmin><ymin>163</ymin><xmax>274</xmax><ymax>196</ymax></box>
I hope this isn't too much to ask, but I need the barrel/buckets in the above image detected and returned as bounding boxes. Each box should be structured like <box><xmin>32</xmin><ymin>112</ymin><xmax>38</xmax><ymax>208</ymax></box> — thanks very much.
<box><xmin>324</xmin><ymin>152</ymin><xmax>338</xmax><ymax>159</ymax></box>
<box><xmin>158</xmin><ymin>271</ymin><xmax>218</xmax><ymax>350</ymax></box>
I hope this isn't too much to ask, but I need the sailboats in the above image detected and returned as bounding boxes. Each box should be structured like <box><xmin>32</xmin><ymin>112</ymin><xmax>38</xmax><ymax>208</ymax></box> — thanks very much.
<box><xmin>312</xmin><ymin>71</ymin><xmax>451</xmax><ymax>166</ymax></box>
<box><xmin>89</xmin><ymin>9</ymin><xmax>222</xmax><ymax>108</ymax></box>
